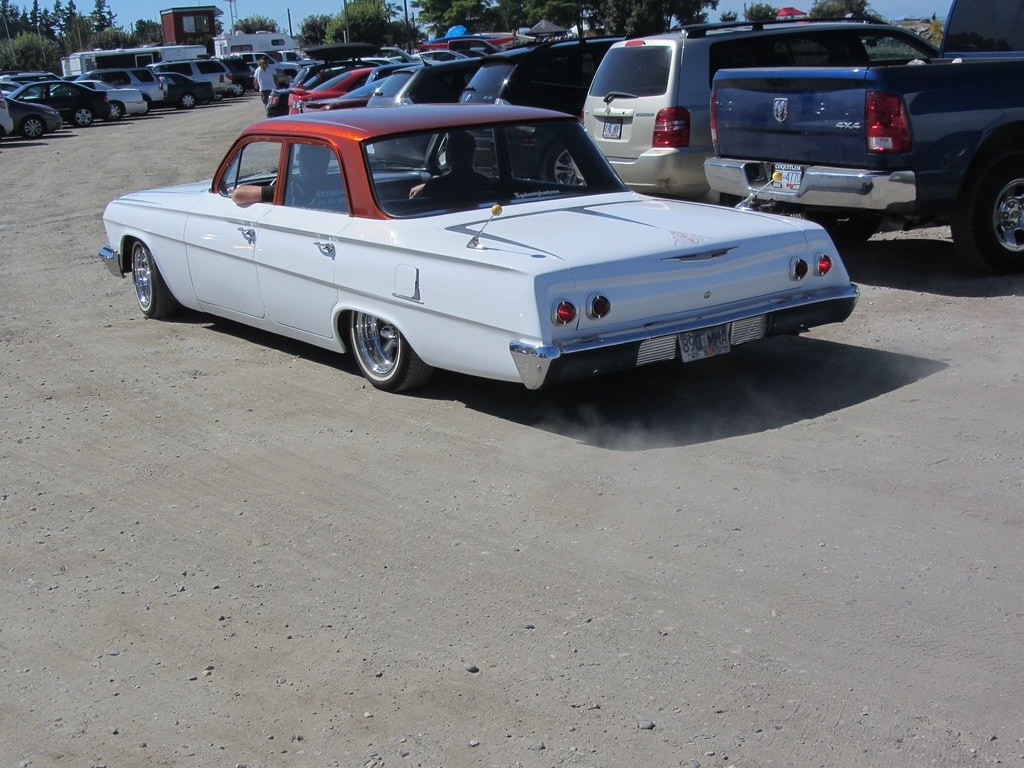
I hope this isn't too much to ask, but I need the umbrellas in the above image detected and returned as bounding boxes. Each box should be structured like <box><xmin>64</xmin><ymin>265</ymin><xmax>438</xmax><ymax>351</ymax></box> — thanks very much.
<box><xmin>445</xmin><ymin>26</ymin><xmax>466</xmax><ymax>38</ymax></box>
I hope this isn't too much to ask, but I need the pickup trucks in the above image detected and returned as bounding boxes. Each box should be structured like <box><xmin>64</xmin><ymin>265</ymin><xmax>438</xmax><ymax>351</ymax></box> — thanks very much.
<box><xmin>701</xmin><ymin>0</ymin><xmax>1023</xmax><ymax>276</ymax></box>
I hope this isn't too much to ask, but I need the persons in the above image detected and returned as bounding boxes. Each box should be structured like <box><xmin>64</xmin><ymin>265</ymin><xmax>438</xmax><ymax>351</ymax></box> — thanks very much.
<box><xmin>408</xmin><ymin>130</ymin><xmax>489</xmax><ymax>199</ymax></box>
<box><xmin>542</xmin><ymin>34</ymin><xmax>579</xmax><ymax>41</ymax></box>
<box><xmin>386</xmin><ymin>39</ymin><xmax>425</xmax><ymax>57</ymax></box>
<box><xmin>254</xmin><ymin>56</ymin><xmax>280</xmax><ymax>108</ymax></box>
<box><xmin>231</xmin><ymin>143</ymin><xmax>331</xmax><ymax>208</ymax></box>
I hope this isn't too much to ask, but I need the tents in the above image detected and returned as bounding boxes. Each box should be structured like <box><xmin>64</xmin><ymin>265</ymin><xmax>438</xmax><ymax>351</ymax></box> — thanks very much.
<box><xmin>525</xmin><ymin>20</ymin><xmax>567</xmax><ymax>42</ymax></box>
<box><xmin>770</xmin><ymin>7</ymin><xmax>807</xmax><ymax>17</ymax></box>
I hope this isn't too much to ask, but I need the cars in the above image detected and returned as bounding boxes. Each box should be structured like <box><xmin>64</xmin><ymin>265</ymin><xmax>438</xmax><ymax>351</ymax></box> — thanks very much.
<box><xmin>582</xmin><ymin>17</ymin><xmax>947</xmax><ymax>203</ymax></box>
<box><xmin>262</xmin><ymin>33</ymin><xmax>625</xmax><ymax>174</ymax></box>
<box><xmin>1</xmin><ymin>29</ymin><xmax>293</xmax><ymax>139</ymax></box>
<box><xmin>103</xmin><ymin>103</ymin><xmax>859</xmax><ymax>399</ymax></box>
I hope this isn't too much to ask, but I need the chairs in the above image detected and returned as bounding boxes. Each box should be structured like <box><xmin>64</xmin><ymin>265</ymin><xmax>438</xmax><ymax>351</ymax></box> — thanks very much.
<box><xmin>826</xmin><ymin>32</ymin><xmax>871</xmax><ymax>64</ymax></box>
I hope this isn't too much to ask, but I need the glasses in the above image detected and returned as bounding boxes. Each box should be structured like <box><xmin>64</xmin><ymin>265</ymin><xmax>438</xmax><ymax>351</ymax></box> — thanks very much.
<box><xmin>296</xmin><ymin>153</ymin><xmax>303</xmax><ymax>161</ymax></box>
